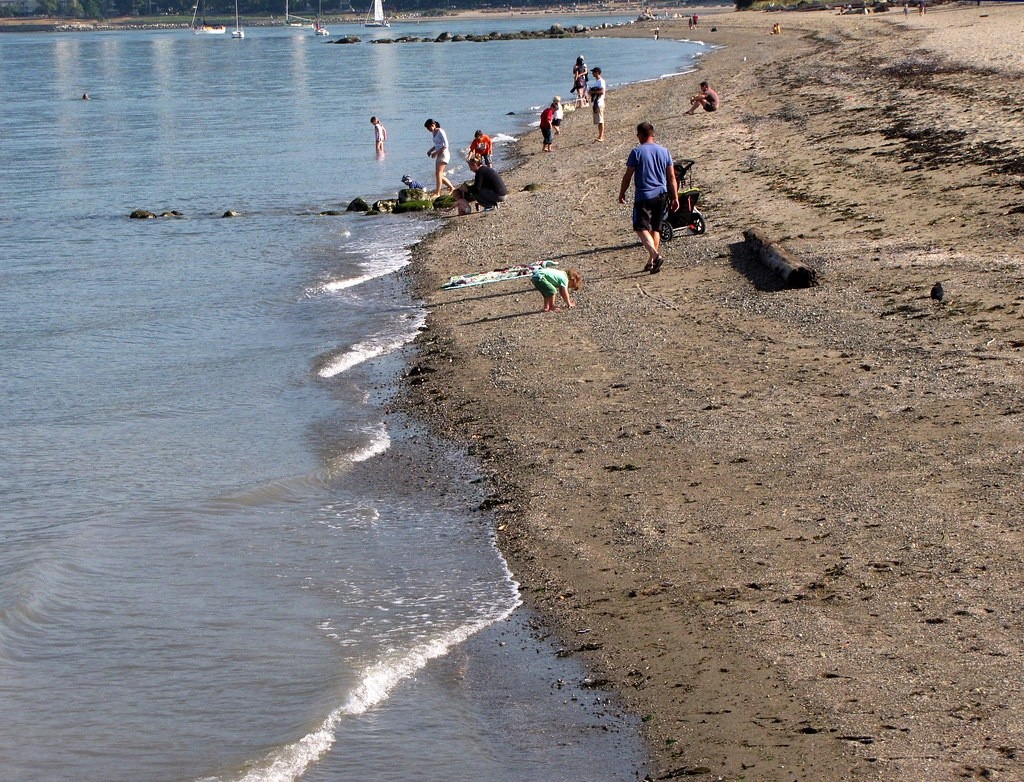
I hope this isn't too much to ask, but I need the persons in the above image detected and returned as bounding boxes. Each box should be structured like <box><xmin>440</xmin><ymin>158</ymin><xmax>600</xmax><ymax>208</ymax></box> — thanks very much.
<box><xmin>531</xmin><ymin>268</ymin><xmax>581</xmax><ymax>311</ymax></box>
<box><xmin>443</xmin><ymin>189</ymin><xmax>471</xmax><ymax>215</ymax></box>
<box><xmin>903</xmin><ymin>4</ymin><xmax>910</xmax><ymax>19</ymax></box>
<box><xmin>773</xmin><ymin>22</ymin><xmax>780</xmax><ymax>34</ymax></box>
<box><xmin>570</xmin><ymin>54</ymin><xmax>589</xmax><ymax>108</ymax></box>
<box><xmin>848</xmin><ymin>3</ymin><xmax>852</xmax><ymax>11</ymax></box>
<box><xmin>424</xmin><ymin>119</ymin><xmax>455</xmax><ymax>199</ymax></box>
<box><xmin>461</xmin><ymin>154</ymin><xmax>508</xmax><ymax>211</ymax></box>
<box><xmin>917</xmin><ymin>0</ymin><xmax>926</xmax><ymax>16</ymax></box>
<box><xmin>402</xmin><ymin>174</ymin><xmax>427</xmax><ymax>193</ymax></box>
<box><xmin>370</xmin><ymin>117</ymin><xmax>386</xmax><ymax>153</ymax></box>
<box><xmin>684</xmin><ymin>82</ymin><xmax>719</xmax><ymax>115</ymax></box>
<box><xmin>654</xmin><ymin>26</ymin><xmax>659</xmax><ymax>40</ymax></box>
<box><xmin>693</xmin><ymin>13</ymin><xmax>698</xmax><ymax>29</ymax></box>
<box><xmin>539</xmin><ymin>103</ymin><xmax>559</xmax><ymax>152</ymax></box>
<box><xmin>689</xmin><ymin>17</ymin><xmax>693</xmax><ymax>30</ymax></box>
<box><xmin>550</xmin><ymin>95</ymin><xmax>563</xmax><ymax>134</ymax></box>
<box><xmin>467</xmin><ymin>130</ymin><xmax>492</xmax><ymax>168</ymax></box>
<box><xmin>83</xmin><ymin>93</ymin><xmax>90</xmax><ymax>99</ymax></box>
<box><xmin>618</xmin><ymin>122</ymin><xmax>679</xmax><ymax>273</ymax></box>
<box><xmin>588</xmin><ymin>67</ymin><xmax>605</xmax><ymax>141</ymax></box>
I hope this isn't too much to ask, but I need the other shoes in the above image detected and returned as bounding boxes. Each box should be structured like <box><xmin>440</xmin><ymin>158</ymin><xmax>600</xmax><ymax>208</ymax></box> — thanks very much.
<box><xmin>482</xmin><ymin>207</ymin><xmax>494</xmax><ymax>211</ymax></box>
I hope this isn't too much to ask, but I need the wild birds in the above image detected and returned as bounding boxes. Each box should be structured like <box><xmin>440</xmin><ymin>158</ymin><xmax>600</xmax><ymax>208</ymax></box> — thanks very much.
<box><xmin>82</xmin><ymin>93</ymin><xmax>91</xmax><ymax>101</ymax></box>
<box><xmin>931</xmin><ymin>281</ymin><xmax>944</xmax><ymax>306</ymax></box>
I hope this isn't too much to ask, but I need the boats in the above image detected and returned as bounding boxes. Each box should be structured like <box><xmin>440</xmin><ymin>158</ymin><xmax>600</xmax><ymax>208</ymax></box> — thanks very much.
<box><xmin>192</xmin><ymin>24</ymin><xmax>227</xmax><ymax>34</ymax></box>
<box><xmin>313</xmin><ymin>18</ymin><xmax>330</xmax><ymax>36</ymax></box>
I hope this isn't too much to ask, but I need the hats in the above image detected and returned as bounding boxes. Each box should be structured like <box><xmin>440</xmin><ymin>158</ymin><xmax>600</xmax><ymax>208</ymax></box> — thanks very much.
<box><xmin>590</xmin><ymin>67</ymin><xmax>601</xmax><ymax>75</ymax></box>
<box><xmin>401</xmin><ymin>174</ymin><xmax>411</xmax><ymax>183</ymax></box>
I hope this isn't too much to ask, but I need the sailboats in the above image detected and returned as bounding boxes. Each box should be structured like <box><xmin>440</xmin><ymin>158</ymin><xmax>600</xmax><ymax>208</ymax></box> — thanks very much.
<box><xmin>232</xmin><ymin>0</ymin><xmax>245</xmax><ymax>38</ymax></box>
<box><xmin>365</xmin><ymin>0</ymin><xmax>391</xmax><ymax>27</ymax></box>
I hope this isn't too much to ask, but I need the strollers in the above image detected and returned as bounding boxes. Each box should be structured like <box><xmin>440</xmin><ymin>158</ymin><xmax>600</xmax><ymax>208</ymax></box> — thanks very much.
<box><xmin>659</xmin><ymin>159</ymin><xmax>707</xmax><ymax>242</ymax></box>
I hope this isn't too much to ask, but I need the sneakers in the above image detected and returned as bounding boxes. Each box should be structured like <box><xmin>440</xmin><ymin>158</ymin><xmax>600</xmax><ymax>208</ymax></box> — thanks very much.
<box><xmin>650</xmin><ymin>255</ymin><xmax>663</xmax><ymax>273</ymax></box>
<box><xmin>645</xmin><ymin>260</ymin><xmax>653</xmax><ymax>270</ymax></box>
